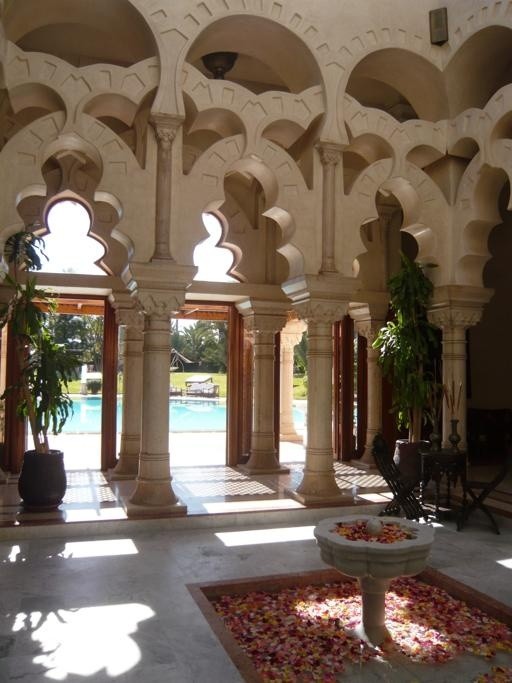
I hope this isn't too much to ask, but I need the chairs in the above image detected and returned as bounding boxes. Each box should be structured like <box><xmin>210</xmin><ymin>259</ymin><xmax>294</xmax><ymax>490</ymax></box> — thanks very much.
<box><xmin>371</xmin><ymin>430</ymin><xmax>430</xmax><ymax>521</ymax></box>
<box><xmin>456</xmin><ymin>464</ymin><xmax>510</xmax><ymax>535</ymax></box>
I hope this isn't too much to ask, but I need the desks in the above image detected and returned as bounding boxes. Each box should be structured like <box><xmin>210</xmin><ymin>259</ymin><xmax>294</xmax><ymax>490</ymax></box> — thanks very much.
<box><xmin>416</xmin><ymin>448</ymin><xmax>467</xmax><ymax>501</ymax></box>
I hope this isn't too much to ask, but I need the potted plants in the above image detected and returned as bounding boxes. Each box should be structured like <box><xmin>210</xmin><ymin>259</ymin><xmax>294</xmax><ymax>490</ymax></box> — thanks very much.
<box><xmin>442</xmin><ymin>367</ymin><xmax>464</xmax><ymax>450</ymax></box>
<box><xmin>0</xmin><ymin>229</ymin><xmax>98</xmax><ymax>508</ymax></box>
<box><xmin>370</xmin><ymin>244</ymin><xmax>445</xmax><ymax>483</ymax></box>
